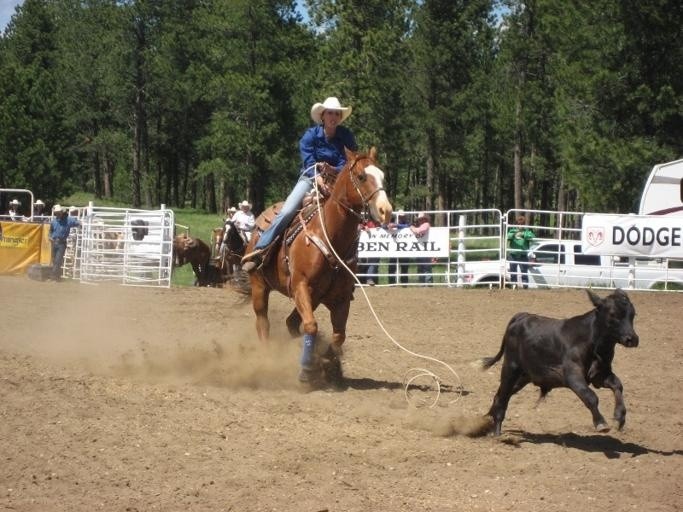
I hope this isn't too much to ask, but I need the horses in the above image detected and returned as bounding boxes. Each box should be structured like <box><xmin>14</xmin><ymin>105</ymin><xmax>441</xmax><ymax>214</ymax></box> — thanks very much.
<box><xmin>214</xmin><ymin>144</ymin><xmax>393</xmax><ymax>395</ymax></box>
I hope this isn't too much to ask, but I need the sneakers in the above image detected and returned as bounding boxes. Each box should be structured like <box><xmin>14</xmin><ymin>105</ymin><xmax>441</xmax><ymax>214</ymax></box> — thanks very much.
<box><xmin>366</xmin><ymin>278</ymin><xmax>376</xmax><ymax>285</ymax></box>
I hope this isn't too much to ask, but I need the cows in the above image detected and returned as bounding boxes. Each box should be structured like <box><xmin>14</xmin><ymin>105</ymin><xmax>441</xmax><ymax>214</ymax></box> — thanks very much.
<box><xmin>175</xmin><ymin>230</ymin><xmax>211</xmax><ymax>286</ymax></box>
<box><xmin>480</xmin><ymin>285</ymin><xmax>640</xmax><ymax>439</ymax></box>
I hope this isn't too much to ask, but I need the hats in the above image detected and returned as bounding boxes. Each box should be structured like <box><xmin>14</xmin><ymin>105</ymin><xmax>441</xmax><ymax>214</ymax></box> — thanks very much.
<box><xmin>238</xmin><ymin>200</ymin><xmax>253</xmax><ymax>210</ymax></box>
<box><xmin>9</xmin><ymin>199</ymin><xmax>22</xmax><ymax>206</ymax></box>
<box><xmin>53</xmin><ymin>204</ymin><xmax>63</xmax><ymax>212</ymax></box>
<box><xmin>311</xmin><ymin>95</ymin><xmax>353</xmax><ymax>125</ymax></box>
<box><xmin>227</xmin><ymin>206</ymin><xmax>238</xmax><ymax>213</ymax></box>
<box><xmin>32</xmin><ymin>200</ymin><xmax>46</xmax><ymax>207</ymax></box>
<box><xmin>414</xmin><ymin>211</ymin><xmax>428</xmax><ymax>222</ymax></box>
<box><xmin>395</xmin><ymin>210</ymin><xmax>406</xmax><ymax>216</ymax></box>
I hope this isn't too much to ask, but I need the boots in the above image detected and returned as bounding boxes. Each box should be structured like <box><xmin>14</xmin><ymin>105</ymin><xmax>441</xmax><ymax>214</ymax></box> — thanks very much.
<box><xmin>242</xmin><ymin>239</ymin><xmax>267</xmax><ymax>272</ymax></box>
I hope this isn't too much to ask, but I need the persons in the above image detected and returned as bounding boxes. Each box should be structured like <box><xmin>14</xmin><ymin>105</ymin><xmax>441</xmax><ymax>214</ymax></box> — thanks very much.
<box><xmin>231</xmin><ymin>200</ymin><xmax>255</xmax><ymax>242</ymax></box>
<box><xmin>225</xmin><ymin>207</ymin><xmax>237</xmax><ymax>221</ymax></box>
<box><xmin>241</xmin><ymin>96</ymin><xmax>359</xmax><ymax>272</ymax></box>
<box><xmin>410</xmin><ymin>213</ymin><xmax>433</xmax><ymax>287</ymax></box>
<box><xmin>507</xmin><ymin>216</ymin><xmax>536</xmax><ymax>289</ymax></box>
<box><xmin>357</xmin><ymin>217</ymin><xmax>382</xmax><ymax>285</ymax></box>
<box><xmin>380</xmin><ymin>209</ymin><xmax>412</xmax><ymax>288</ymax></box>
<box><xmin>47</xmin><ymin>206</ymin><xmax>80</xmax><ymax>282</ymax></box>
<box><xmin>3</xmin><ymin>199</ymin><xmax>78</xmax><ymax>222</ymax></box>
